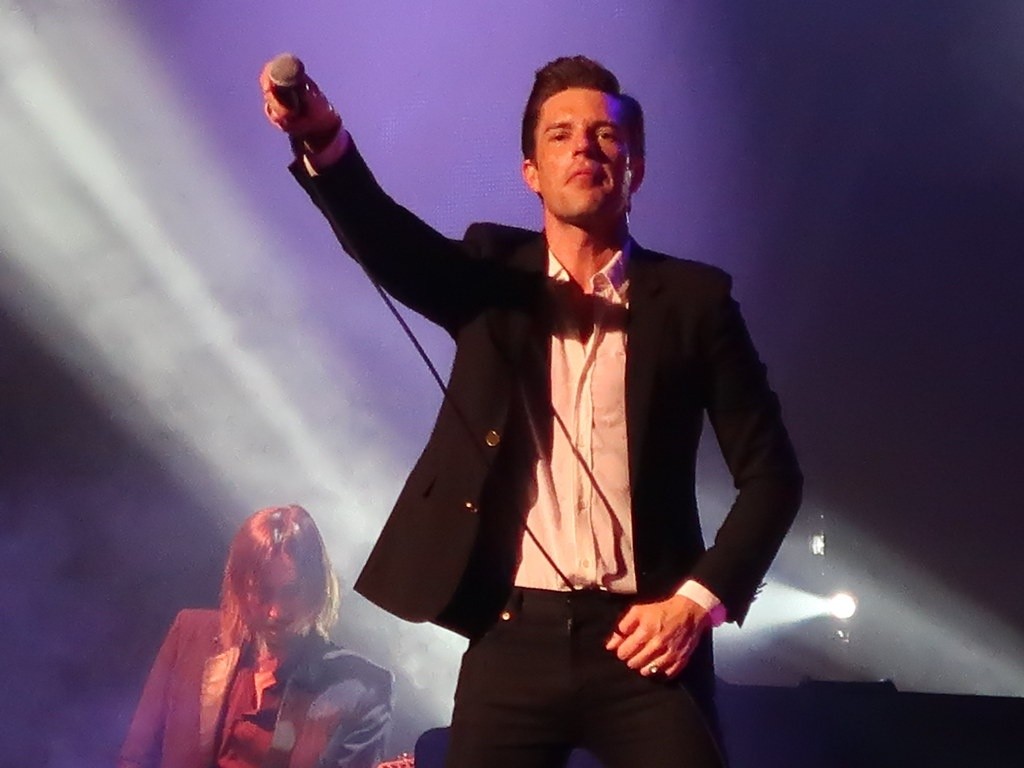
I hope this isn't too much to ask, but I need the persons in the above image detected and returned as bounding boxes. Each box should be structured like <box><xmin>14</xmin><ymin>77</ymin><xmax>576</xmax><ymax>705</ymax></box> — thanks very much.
<box><xmin>259</xmin><ymin>53</ymin><xmax>806</xmax><ymax>768</ymax></box>
<box><xmin>113</xmin><ymin>502</ymin><xmax>395</xmax><ymax>767</ymax></box>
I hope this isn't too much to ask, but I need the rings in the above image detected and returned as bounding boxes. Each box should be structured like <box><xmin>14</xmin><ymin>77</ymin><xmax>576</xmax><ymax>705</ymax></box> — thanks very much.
<box><xmin>648</xmin><ymin>661</ymin><xmax>659</xmax><ymax>673</ymax></box>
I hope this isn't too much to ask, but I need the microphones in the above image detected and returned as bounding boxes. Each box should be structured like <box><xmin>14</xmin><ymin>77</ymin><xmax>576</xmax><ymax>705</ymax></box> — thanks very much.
<box><xmin>268</xmin><ymin>52</ymin><xmax>306</xmax><ymax>161</ymax></box>
